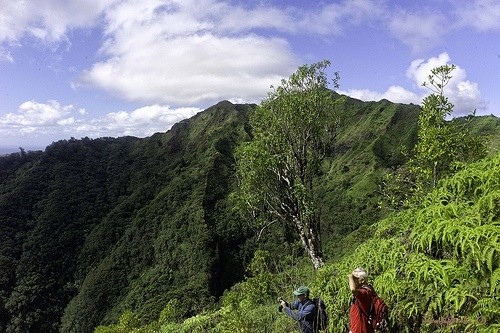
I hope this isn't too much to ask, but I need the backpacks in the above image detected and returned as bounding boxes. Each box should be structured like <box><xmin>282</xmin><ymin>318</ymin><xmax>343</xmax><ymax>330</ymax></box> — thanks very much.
<box><xmin>356</xmin><ymin>283</ymin><xmax>391</xmax><ymax>333</ymax></box>
<box><xmin>300</xmin><ymin>298</ymin><xmax>327</xmax><ymax>332</ymax></box>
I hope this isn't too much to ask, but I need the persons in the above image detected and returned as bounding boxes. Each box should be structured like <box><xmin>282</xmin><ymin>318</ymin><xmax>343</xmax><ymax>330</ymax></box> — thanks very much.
<box><xmin>279</xmin><ymin>286</ymin><xmax>318</xmax><ymax>333</ymax></box>
<box><xmin>347</xmin><ymin>269</ymin><xmax>376</xmax><ymax>333</ymax></box>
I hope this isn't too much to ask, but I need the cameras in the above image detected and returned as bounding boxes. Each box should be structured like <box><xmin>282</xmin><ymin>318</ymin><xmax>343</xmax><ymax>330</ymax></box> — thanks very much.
<box><xmin>277</xmin><ymin>297</ymin><xmax>282</xmax><ymax>303</ymax></box>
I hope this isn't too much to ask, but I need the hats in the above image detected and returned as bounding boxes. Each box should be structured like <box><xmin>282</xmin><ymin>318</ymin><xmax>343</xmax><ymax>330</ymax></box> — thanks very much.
<box><xmin>348</xmin><ymin>267</ymin><xmax>369</xmax><ymax>280</ymax></box>
<box><xmin>291</xmin><ymin>286</ymin><xmax>309</xmax><ymax>296</ymax></box>
